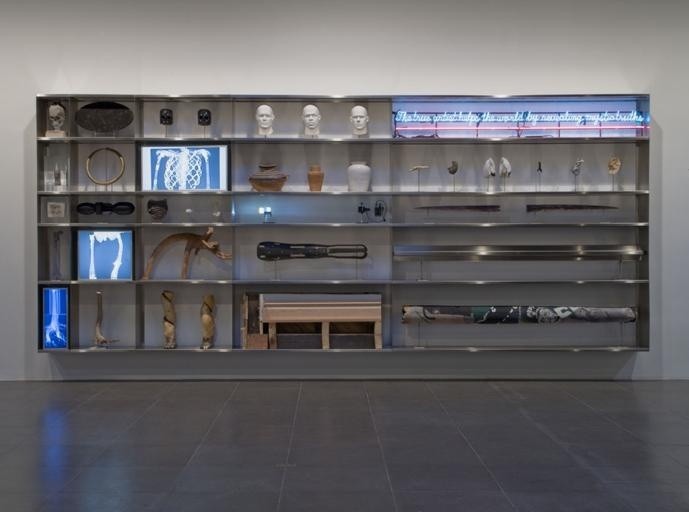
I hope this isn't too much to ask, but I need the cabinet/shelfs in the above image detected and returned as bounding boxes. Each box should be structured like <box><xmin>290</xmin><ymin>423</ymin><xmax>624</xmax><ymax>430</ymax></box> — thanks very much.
<box><xmin>36</xmin><ymin>94</ymin><xmax>651</xmax><ymax>352</ymax></box>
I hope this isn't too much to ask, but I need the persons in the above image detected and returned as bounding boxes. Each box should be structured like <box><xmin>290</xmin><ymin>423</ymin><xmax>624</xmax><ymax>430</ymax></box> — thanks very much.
<box><xmin>256</xmin><ymin>105</ymin><xmax>274</xmax><ymax>136</ymax></box>
<box><xmin>350</xmin><ymin>106</ymin><xmax>369</xmax><ymax>135</ymax></box>
<box><xmin>301</xmin><ymin>105</ymin><xmax>321</xmax><ymax>136</ymax></box>
